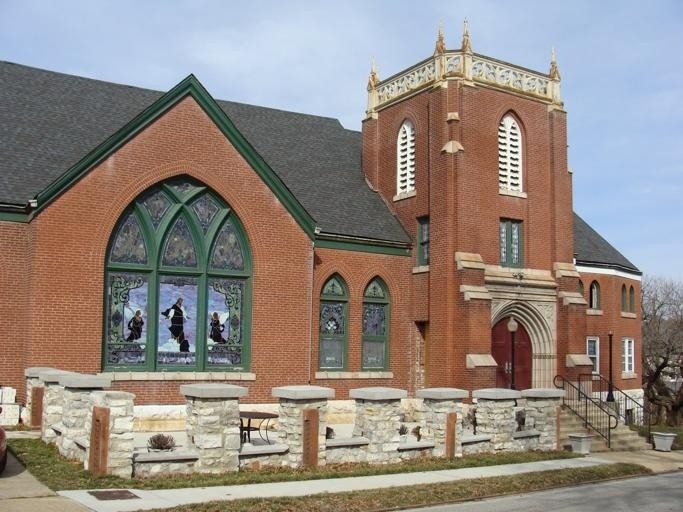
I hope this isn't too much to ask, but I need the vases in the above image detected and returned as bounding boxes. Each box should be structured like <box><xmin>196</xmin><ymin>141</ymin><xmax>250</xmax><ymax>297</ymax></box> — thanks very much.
<box><xmin>649</xmin><ymin>430</ymin><xmax>677</xmax><ymax>453</ymax></box>
<box><xmin>566</xmin><ymin>432</ymin><xmax>596</xmax><ymax>456</ymax></box>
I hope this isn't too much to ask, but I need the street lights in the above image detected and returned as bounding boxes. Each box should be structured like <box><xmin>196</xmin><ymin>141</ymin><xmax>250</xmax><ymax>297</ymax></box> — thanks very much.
<box><xmin>507</xmin><ymin>317</ymin><xmax>518</xmax><ymax>389</ymax></box>
<box><xmin>607</xmin><ymin>330</ymin><xmax>615</xmax><ymax>401</ymax></box>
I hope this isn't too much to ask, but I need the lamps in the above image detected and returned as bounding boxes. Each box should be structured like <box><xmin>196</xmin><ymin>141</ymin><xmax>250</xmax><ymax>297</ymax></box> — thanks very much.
<box><xmin>506</xmin><ymin>314</ymin><xmax>519</xmax><ymax>332</ymax></box>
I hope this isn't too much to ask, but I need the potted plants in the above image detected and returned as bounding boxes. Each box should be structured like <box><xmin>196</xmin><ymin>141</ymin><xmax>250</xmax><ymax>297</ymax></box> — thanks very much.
<box><xmin>145</xmin><ymin>428</ymin><xmax>178</xmax><ymax>454</ymax></box>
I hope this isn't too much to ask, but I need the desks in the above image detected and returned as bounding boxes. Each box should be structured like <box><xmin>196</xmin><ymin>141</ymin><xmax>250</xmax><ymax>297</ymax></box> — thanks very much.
<box><xmin>239</xmin><ymin>411</ymin><xmax>280</xmax><ymax>444</ymax></box>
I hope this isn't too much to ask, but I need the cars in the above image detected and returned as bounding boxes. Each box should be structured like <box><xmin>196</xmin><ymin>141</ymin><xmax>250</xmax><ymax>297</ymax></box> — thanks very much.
<box><xmin>0</xmin><ymin>406</ymin><xmax>7</xmax><ymax>473</ymax></box>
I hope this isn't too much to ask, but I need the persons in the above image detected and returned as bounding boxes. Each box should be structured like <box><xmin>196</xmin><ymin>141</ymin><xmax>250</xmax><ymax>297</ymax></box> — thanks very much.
<box><xmin>208</xmin><ymin>311</ymin><xmax>227</xmax><ymax>344</ymax></box>
<box><xmin>124</xmin><ymin>310</ymin><xmax>144</xmax><ymax>341</ymax></box>
<box><xmin>160</xmin><ymin>298</ymin><xmax>190</xmax><ymax>352</ymax></box>
